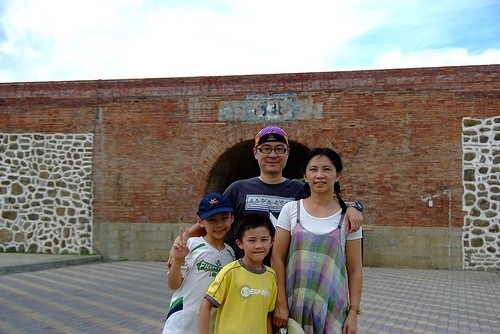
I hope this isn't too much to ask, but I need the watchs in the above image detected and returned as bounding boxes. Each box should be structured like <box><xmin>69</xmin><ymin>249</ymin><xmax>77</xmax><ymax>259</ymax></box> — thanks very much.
<box><xmin>346</xmin><ymin>201</ymin><xmax>364</xmax><ymax>212</ymax></box>
<box><xmin>346</xmin><ymin>304</ymin><xmax>361</xmax><ymax>314</ymax></box>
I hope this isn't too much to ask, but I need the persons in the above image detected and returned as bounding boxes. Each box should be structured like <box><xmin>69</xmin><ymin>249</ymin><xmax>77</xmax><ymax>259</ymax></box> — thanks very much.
<box><xmin>198</xmin><ymin>211</ymin><xmax>279</xmax><ymax>333</ymax></box>
<box><xmin>162</xmin><ymin>192</ymin><xmax>239</xmax><ymax>334</ymax></box>
<box><xmin>270</xmin><ymin>148</ymin><xmax>365</xmax><ymax>334</ymax></box>
<box><xmin>167</xmin><ymin>126</ymin><xmax>364</xmax><ymax>274</ymax></box>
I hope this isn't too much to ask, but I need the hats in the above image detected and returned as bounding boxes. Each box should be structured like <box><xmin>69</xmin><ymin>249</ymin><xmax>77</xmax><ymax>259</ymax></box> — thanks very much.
<box><xmin>255</xmin><ymin>126</ymin><xmax>289</xmax><ymax>147</ymax></box>
<box><xmin>198</xmin><ymin>193</ymin><xmax>233</xmax><ymax>220</ymax></box>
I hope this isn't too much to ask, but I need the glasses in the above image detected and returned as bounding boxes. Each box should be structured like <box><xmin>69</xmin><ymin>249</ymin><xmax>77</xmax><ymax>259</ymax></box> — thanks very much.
<box><xmin>256</xmin><ymin>145</ymin><xmax>287</xmax><ymax>153</ymax></box>
